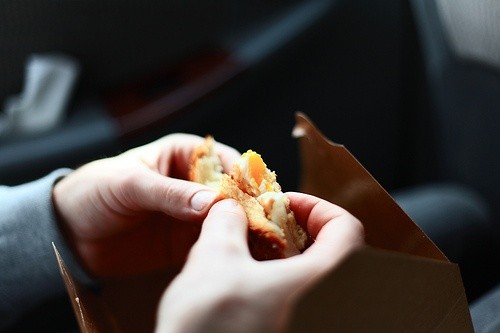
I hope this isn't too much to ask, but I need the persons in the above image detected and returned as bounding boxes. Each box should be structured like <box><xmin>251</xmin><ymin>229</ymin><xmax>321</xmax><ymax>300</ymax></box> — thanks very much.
<box><xmin>0</xmin><ymin>132</ymin><xmax>365</xmax><ymax>332</ymax></box>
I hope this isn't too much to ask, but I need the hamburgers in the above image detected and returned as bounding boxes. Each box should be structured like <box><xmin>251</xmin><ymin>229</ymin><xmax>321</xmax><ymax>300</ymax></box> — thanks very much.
<box><xmin>188</xmin><ymin>135</ymin><xmax>308</xmax><ymax>260</ymax></box>
